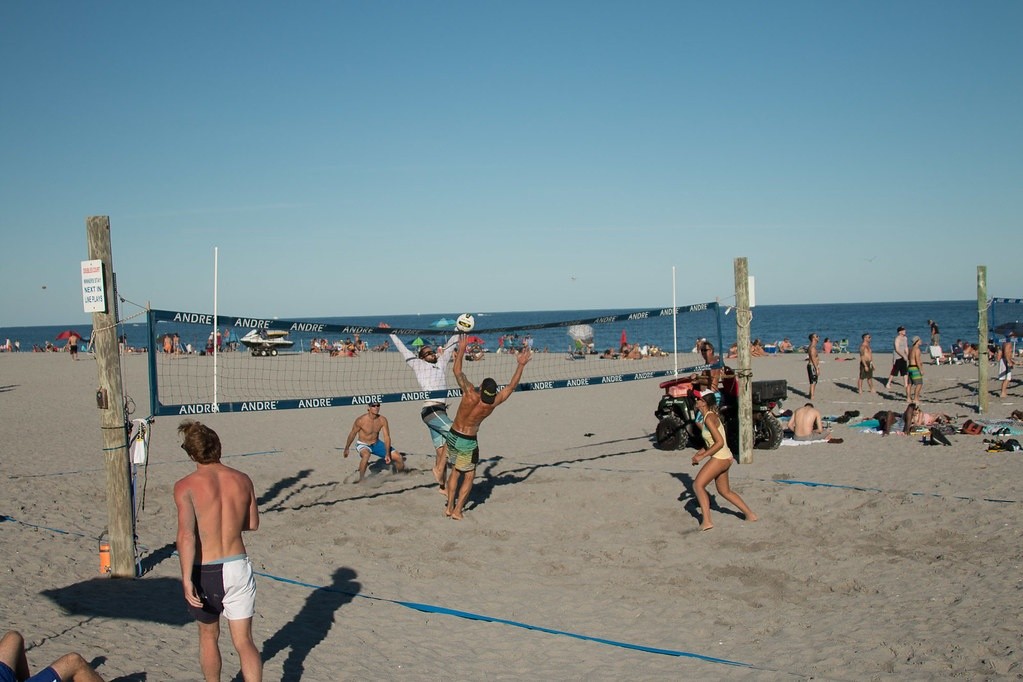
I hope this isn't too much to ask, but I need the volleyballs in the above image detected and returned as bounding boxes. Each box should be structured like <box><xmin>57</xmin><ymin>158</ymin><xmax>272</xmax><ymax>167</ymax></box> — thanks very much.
<box><xmin>456</xmin><ymin>313</ymin><xmax>475</xmax><ymax>332</ymax></box>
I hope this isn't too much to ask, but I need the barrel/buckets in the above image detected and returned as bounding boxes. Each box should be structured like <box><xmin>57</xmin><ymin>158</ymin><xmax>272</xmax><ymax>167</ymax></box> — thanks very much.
<box><xmin>100</xmin><ymin>545</ymin><xmax>111</xmax><ymax>575</ymax></box>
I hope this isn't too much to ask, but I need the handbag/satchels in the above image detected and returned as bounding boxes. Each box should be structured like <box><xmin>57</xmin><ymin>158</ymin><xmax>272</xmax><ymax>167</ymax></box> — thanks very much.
<box><xmin>962</xmin><ymin>420</ymin><xmax>983</xmax><ymax>435</ymax></box>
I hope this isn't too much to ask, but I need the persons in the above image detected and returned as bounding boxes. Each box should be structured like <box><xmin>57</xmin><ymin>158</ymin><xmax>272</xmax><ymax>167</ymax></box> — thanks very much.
<box><xmin>752</xmin><ymin>318</ymin><xmax>1013</xmax><ymax>441</ymax></box>
<box><xmin>15</xmin><ymin>339</ymin><xmax>20</xmax><ymax>352</ymax></box>
<box><xmin>68</xmin><ymin>332</ymin><xmax>79</xmax><ymax>360</ymax></box>
<box><xmin>0</xmin><ymin>630</ymin><xmax>104</xmax><ymax>682</ymax></box>
<box><xmin>311</xmin><ymin>333</ymin><xmax>389</xmax><ymax>358</ymax></box>
<box><xmin>163</xmin><ymin>333</ymin><xmax>180</xmax><ymax>356</ymax></box>
<box><xmin>692</xmin><ymin>393</ymin><xmax>759</xmax><ymax>530</ymax></box>
<box><xmin>469</xmin><ymin>341</ymin><xmax>484</xmax><ymax>360</ymax></box>
<box><xmin>173</xmin><ymin>422</ymin><xmax>263</xmax><ymax>682</ymax></box>
<box><xmin>497</xmin><ymin>332</ymin><xmax>533</xmax><ymax>355</ymax></box>
<box><xmin>208</xmin><ymin>327</ymin><xmax>229</xmax><ymax>351</ymax></box>
<box><xmin>445</xmin><ymin>333</ymin><xmax>532</xmax><ymax>520</ymax></box>
<box><xmin>380</xmin><ymin>322</ymin><xmax>460</xmax><ymax>495</ymax></box>
<box><xmin>31</xmin><ymin>344</ymin><xmax>43</xmax><ymax>352</ymax></box>
<box><xmin>696</xmin><ymin>338</ymin><xmax>721</xmax><ymax>429</ymax></box>
<box><xmin>6</xmin><ymin>337</ymin><xmax>11</xmax><ymax>352</ymax></box>
<box><xmin>344</xmin><ymin>402</ymin><xmax>404</xmax><ymax>481</ymax></box>
<box><xmin>118</xmin><ymin>333</ymin><xmax>127</xmax><ymax>355</ymax></box>
<box><xmin>575</xmin><ymin>339</ymin><xmax>667</xmax><ymax>360</ymax></box>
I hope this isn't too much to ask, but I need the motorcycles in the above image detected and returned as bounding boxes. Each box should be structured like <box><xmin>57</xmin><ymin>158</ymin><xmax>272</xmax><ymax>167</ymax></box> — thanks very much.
<box><xmin>656</xmin><ymin>366</ymin><xmax>789</xmax><ymax>451</ymax></box>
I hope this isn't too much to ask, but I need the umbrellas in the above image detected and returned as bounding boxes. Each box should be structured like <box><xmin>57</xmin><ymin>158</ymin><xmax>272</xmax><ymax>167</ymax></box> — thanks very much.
<box><xmin>990</xmin><ymin>322</ymin><xmax>1023</xmax><ymax>337</ymax></box>
<box><xmin>55</xmin><ymin>328</ymin><xmax>81</xmax><ymax>340</ymax></box>
<box><xmin>467</xmin><ymin>335</ymin><xmax>485</xmax><ymax>344</ymax></box>
<box><xmin>405</xmin><ymin>336</ymin><xmax>432</xmax><ymax>345</ymax></box>
<box><xmin>566</xmin><ymin>324</ymin><xmax>595</xmax><ymax>340</ymax></box>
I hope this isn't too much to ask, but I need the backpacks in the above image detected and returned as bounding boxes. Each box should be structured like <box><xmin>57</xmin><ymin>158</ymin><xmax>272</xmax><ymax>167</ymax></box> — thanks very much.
<box><xmin>929</xmin><ymin>426</ymin><xmax>952</xmax><ymax>446</ymax></box>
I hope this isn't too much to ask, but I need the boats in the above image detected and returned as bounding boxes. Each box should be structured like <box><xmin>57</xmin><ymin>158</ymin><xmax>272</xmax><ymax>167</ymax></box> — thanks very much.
<box><xmin>239</xmin><ymin>329</ymin><xmax>294</xmax><ymax>349</ymax></box>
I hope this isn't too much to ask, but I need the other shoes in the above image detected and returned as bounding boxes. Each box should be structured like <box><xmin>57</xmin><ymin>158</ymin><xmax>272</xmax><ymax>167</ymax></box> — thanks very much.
<box><xmin>988</xmin><ymin>444</ymin><xmax>1006</xmax><ymax>453</ymax></box>
<box><xmin>983</xmin><ymin>438</ymin><xmax>997</xmax><ymax>445</ymax></box>
<box><xmin>998</xmin><ymin>428</ymin><xmax>1011</xmax><ymax>436</ymax></box>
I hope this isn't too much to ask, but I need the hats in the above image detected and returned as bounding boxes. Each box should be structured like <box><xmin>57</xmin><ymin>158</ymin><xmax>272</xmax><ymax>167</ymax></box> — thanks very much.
<box><xmin>897</xmin><ymin>326</ymin><xmax>905</xmax><ymax>333</ymax></box>
<box><xmin>480</xmin><ymin>378</ymin><xmax>496</xmax><ymax>403</ymax></box>
<box><xmin>911</xmin><ymin>335</ymin><xmax>919</xmax><ymax>346</ymax></box>
<box><xmin>693</xmin><ymin>389</ymin><xmax>717</xmax><ymax>406</ymax></box>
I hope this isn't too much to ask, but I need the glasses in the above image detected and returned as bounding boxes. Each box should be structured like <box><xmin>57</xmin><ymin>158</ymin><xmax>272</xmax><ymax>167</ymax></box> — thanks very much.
<box><xmin>696</xmin><ymin>395</ymin><xmax>707</xmax><ymax>403</ymax></box>
<box><xmin>423</xmin><ymin>350</ymin><xmax>436</xmax><ymax>355</ymax></box>
<box><xmin>370</xmin><ymin>404</ymin><xmax>380</xmax><ymax>408</ymax></box>
<box><xmin>701</xmin><ymin>348</ymin><xmax>714</xmax><ymax>352</ymax></box>
